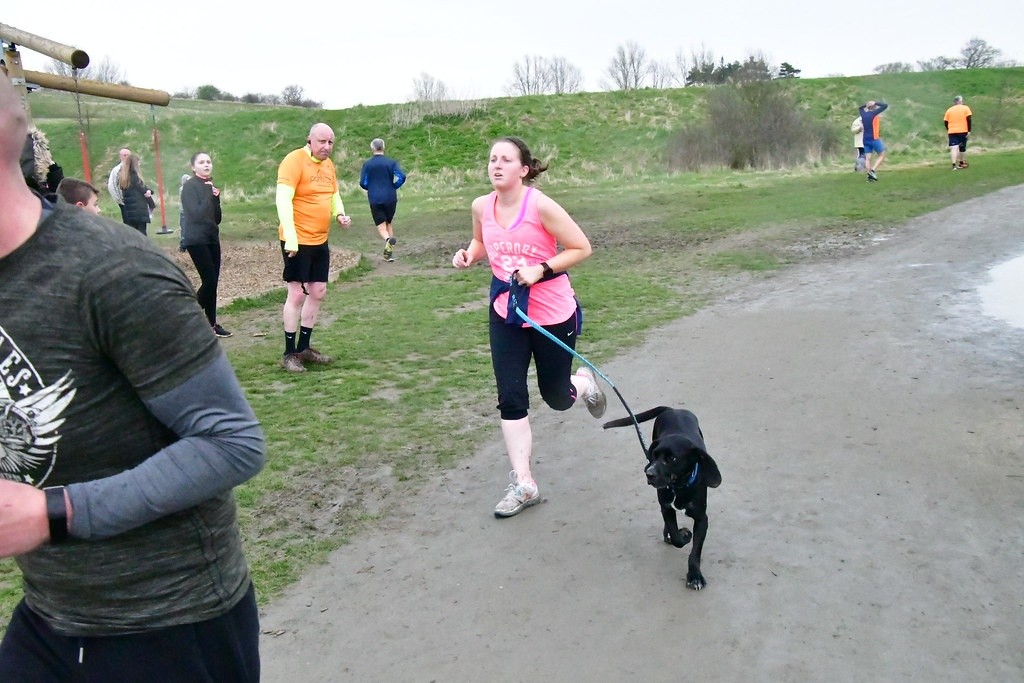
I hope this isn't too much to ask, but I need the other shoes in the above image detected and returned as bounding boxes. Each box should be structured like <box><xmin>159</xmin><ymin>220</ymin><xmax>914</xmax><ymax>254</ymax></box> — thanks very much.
<box><xmin>960</xmin><ymin>162</ymin><xmax>968</xmax><ymax>167</ymax></box>
<box><xmin>953</xmin><ymin>167</ymin><xmax>957</xmax><ymax>171</ymax></box>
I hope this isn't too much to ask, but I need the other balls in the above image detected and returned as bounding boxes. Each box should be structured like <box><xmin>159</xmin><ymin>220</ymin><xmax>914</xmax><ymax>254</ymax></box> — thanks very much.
<box><xmin>962</xmin><ymin>160</ymin><xmax>969</xmax><ymax>166</ymax></box>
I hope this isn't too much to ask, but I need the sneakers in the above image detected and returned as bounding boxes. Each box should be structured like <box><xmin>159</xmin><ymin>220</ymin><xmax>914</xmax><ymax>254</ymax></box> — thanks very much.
<box><xmin>494</xmin><ymin>480</ymin><xmax>539</xmax><ymax>516</ymax></box>
<box><xmin>383</xmin><ymin>237</ymin><xmax>395</xmax><ymax>258</ymax></box>
<box><xmin>281</xmin><ymin>353</ymin><xmax>306</xmax><ymax>374</ymax></box>
<box><xmin>578</xmin><ymin>366</ymin><xmax>606</xmax><ymax>419</ymax></box>
<box><xmin>299</xmin><ymin>347</ymin><xmax>329</xmax><ymax>363</ymax></box>
<box><xmin>867</xmin><ymin>170</ymin><xmax>878</xmax><ymax>181</ymax></box>
<box><xmin>384</xmin><ymin>258</ymin><xmax>394</xmax><ymax>262</ymax></box>
<box><xmin>213</xmin><ymin>324</ymin><xmax>232</xmax><ymax>338</ymax></box>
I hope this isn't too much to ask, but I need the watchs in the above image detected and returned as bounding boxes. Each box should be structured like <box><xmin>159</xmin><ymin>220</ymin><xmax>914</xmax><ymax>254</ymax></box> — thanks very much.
<box><xmin>540</xmin><ymin>262</ymin><xmax>553</xmax><ymax>278</ymax></box>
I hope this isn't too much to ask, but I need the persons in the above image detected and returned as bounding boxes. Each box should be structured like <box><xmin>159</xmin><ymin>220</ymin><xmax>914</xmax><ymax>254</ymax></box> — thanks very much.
<box><xmin>55</xmin><ymin>176</ymin><xmax>102</xmax><ymax>218</ymax></box>
<box><xmin>275</xmin><ymin>123</ymin><xmax>352</xmax><ymax>372</ymax></box>
<box><xmin>177</xmin><ymin>174</ymin><xmax>191</xmax><ymax>251</ymax></box>
<box><xmin>858</xmin><ymin>101</ymin><xmax>888</xmax><ymax>182</ymax></box>
<box><xmin>451</xmin><ymin>136</ymin><xmax>607</xmax><ymax>517</ymax></box>
<box><xmin>181</xmin><ymin>152</ymin><xmax>234</xmax><ymax>338</ymax></box>
<box><xmin>108</xmin><ymin>149</ymin><xmax>131</xmax><ymax>223</ymax></box>
<box><xmin>0</xmin><ymin>67</ymin><xmax>266</xmax><ymax>683</ymax></box>
<box><xmin>944</xmin><ymin>96</ymin><xmax>972</xmax><ymax>171</ymax></box>
<box><xmin>117</xmin><ymin>154</ymin><xmax>157</xmax><ymax>236</ymax></box>
<box><xmin>359</xmin><ymin>138</ymin><xmax>406</xmax><ymax>262</ymax></box>
<box><xmin>851</xmin><ymin>115</ymin><xmax>864</xmax><ymax>172</ymax></box>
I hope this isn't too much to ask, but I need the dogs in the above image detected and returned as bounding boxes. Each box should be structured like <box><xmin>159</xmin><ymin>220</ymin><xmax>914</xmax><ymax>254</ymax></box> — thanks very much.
<box><xmin>603</xmin><ymin>405</ymin><xmax>722</xmax><ymax>590</ymax></box>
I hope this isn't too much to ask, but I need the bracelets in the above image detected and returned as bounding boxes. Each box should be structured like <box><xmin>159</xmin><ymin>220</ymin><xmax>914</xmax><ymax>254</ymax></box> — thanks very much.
<box><xmin>44</xmin><ymin>487</ymin><xmax>69</xmax><ymax>543</ymax></box>
